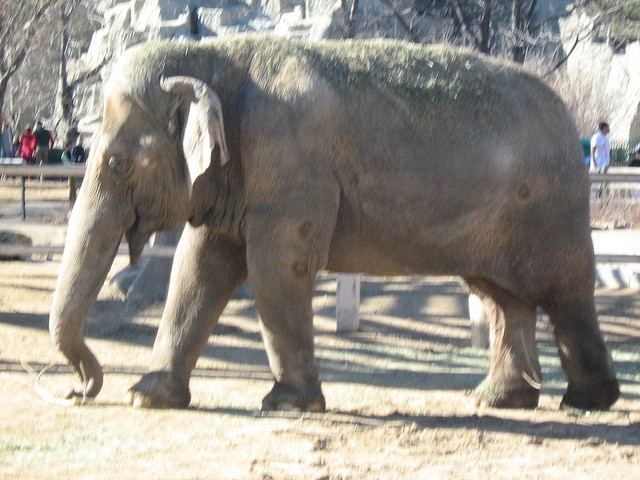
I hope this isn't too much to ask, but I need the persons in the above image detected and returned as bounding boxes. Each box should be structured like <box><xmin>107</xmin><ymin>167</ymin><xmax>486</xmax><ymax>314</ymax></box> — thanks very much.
<box><xmin>31</xmin><ymin>122</ymin><xmax>54</xmax><ymax>182</ymax></box>
<box><xmin>0</xmin><ymin>120</ymin><xmax>12</xmax><ymax>180</ymax></box>
<box><xmin>15</xmin><ymin>127</ymin><xmax>36</xmax><ymax>179</ymax></box>
<box><xmin>590</xmin><ymin>122</ymin><xmax>612</xmax><ymax>206</ymax></box>
<box><xmin>8</xmin><ymin>133</ymin><xmax>22</xmax><ymax>183</ymax></box>
<box><xmin>67</xmin><ymin>135</ymin><xmax>85</xmax><ymax>198</ymax></box>
<box><xmin>62</xmin><ymin>118</ymin><xmax>79</xmax><ymax>182</ymax></box>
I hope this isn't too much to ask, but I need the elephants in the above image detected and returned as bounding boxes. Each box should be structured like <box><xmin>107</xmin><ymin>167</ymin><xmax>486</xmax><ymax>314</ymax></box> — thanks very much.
<box><xmin>48</xmin><ymin>31</ymin><xmax>621</xmax><ymax>417</ymax></box>
